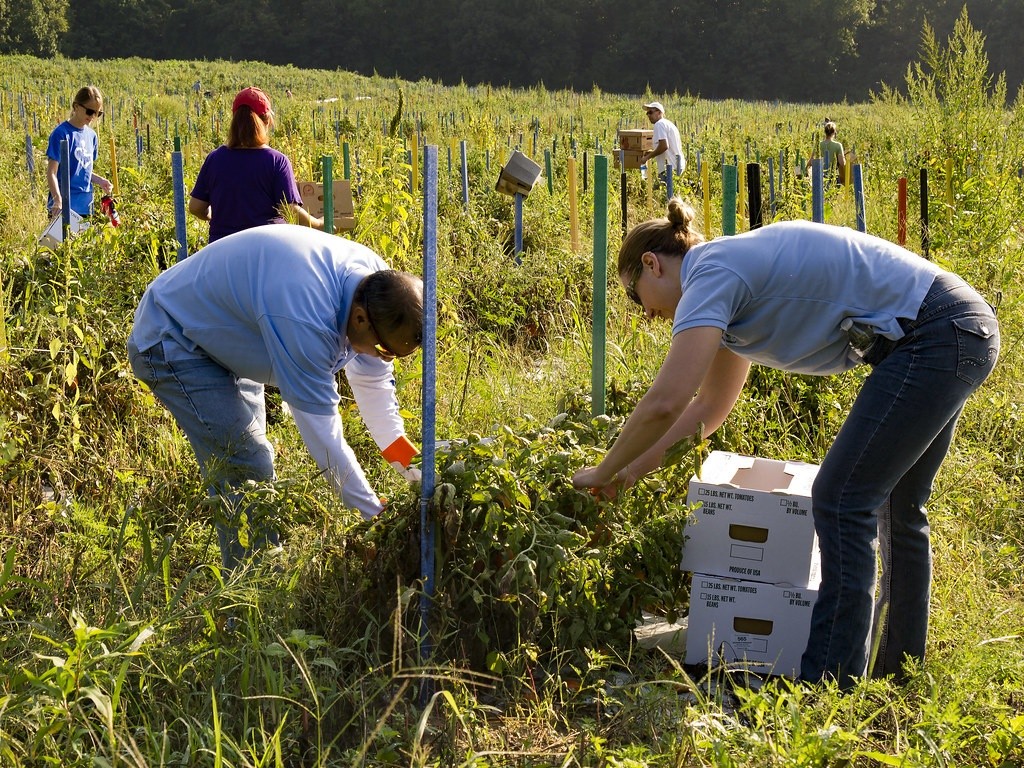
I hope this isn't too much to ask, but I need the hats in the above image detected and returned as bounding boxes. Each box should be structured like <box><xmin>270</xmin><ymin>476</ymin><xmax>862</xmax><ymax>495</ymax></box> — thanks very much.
<box><xmin>233</xmin><ymin>86</ymin><xmax>272</xmax><ymax>118</ymax></box>
<box><xmin>642</xmin><ymin>102</ymin><xmax>665</xmax><ymax>114</ymax></box>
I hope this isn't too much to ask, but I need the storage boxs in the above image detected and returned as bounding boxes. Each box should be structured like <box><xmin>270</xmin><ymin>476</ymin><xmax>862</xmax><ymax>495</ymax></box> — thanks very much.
<box><xmin>684</xmin><ymin>536</ymin><xmax>822</xmax><ymax>678</ymax></box>
<box><xmin>840</xmin><ymin>151</ymin><xmax>859</xmax><ymax>187</ymax></box>
<box><xmin>613</xmin><ymin>149</ymin><xmax>651</xmax><ymax>168</ymax></box>
<box><xmin>296</xmin><ymin>181</ymin><xmax>356</xmax><ymax>234</ymax></box>
<box><xmin>495</xmin><ymin>151</ymin><xmax>543</xmax><ymax>200</ymax></box>
<box><xmin>39</xmin><ymin>207</ymin><xmax>93</xmax><ymax>250</ymax></box>
<box><xmin>618</xmin><ymin>129</ymin><xmax>655</xmax><ymax>151</ymax></box>
<box><xmin>676</xmin><ymin>450</ymin><xmax>822</xmax><ymax>587</ymax></box>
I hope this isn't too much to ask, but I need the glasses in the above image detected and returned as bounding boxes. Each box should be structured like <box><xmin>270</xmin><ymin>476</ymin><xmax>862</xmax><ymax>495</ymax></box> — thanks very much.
<box><xmin>74</xmin><ymin>102</ymin><xmax>102</xmax><ymax>118</ymax></box>
<box><xmin>646</xmin><ymin>110</ymin><xmax>657</xmax><ymax>115</ymax></box>
<box><xmin>626</xmin><ymin>245</ymin><xmax>661</xmax><ymax>306</ymax></box>
<box><xmin>366</xmin><ymin>291</ymin><xmax>416</xmax><ymax>359</ymax></box>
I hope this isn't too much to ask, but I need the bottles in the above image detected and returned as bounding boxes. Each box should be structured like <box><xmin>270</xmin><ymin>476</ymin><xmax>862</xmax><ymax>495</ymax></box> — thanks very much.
<box><xmin>101</xmin><ymin>195</ymin><xmax>121</xmax><ymax>228</ymax></box>
<box><xmin>840</xmin><ymin>318</ymin><xmax>876</xmax><ymax>356</ymax></box>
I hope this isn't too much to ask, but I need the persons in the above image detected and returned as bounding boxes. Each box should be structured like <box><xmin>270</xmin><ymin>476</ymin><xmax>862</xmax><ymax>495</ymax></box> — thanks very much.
<box><xmin>124</xmin><ymin>221</ymin><xmax>424</xmax><ymax>581</ymax></box>
<box><xmin>45</xmin><ymin>87</ymin><xmax>115</xmax><ymax>245</ymax></box>
<box><xmin>575</xmin><ymin>196</ymin><xmax>1002</xmax><ymax>688</ymax></box>
<box><xmin>806</xmin><ymin>118</ymin><xmax>846</xmax><ymax>186</ymax></box>
<box><xmin>191</xmin><ymin>88</ymin><xmax>326</xmax><ymax>245</ymax></box>
<box><xmin>640</xmin><ymin>102</ymin><xmax>687</xmax><ymax>194</ymax></box>
